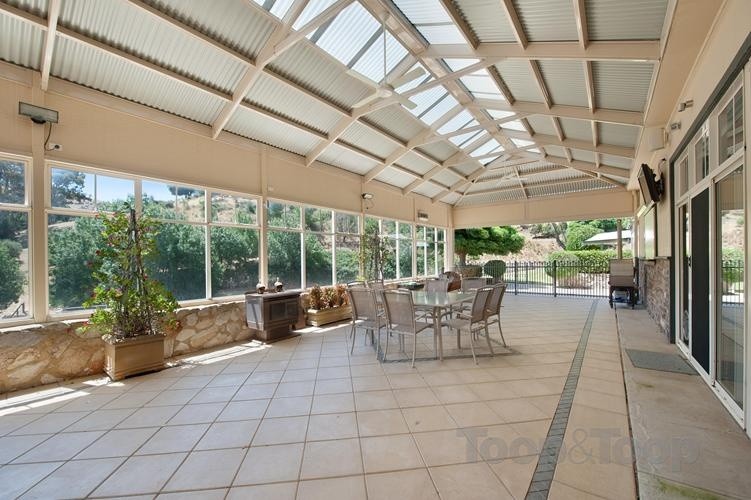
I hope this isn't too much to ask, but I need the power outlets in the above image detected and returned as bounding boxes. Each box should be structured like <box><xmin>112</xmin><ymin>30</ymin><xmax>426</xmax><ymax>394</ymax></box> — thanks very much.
<box><xmin>49</xmin><ymin>143</ymin><xmax>62</xmax><ymax>151</ymax></box>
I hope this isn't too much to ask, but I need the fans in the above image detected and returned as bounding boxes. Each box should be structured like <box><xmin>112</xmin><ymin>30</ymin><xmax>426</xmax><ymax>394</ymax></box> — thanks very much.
<box><xmin>345</xmin><ymin>11</ymin><xmax>426</xmax><ymax>110</ymax></box>
<box><xmin>493</xmin><ymin>144</ymin><xmax>527</xmax><ymax>185</ymax></box>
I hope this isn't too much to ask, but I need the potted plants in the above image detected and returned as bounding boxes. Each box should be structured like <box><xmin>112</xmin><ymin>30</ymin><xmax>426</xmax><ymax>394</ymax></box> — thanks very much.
<box><xmin>307</xmin><ymin>285</ymin><xmax>353</xmax><ymax>326</ymax></box>
<box><xmin>66</xmin><ymin>201</ymin><xmax>184</xmax><ymax>381</ymax></box>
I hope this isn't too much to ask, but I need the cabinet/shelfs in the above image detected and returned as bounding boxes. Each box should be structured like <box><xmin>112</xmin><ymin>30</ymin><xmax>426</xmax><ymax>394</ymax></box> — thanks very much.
<box><xmin>246</xmin><ymin>291</ymin><xmax>302</xmax><ymax>343</ymax></box>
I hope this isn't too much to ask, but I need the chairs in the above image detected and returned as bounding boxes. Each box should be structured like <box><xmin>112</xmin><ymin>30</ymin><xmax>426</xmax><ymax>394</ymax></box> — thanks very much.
<box><xmin>348</xmin><ymin>273</ymin><xmax>513</xmax><ymax>369</ymax></box>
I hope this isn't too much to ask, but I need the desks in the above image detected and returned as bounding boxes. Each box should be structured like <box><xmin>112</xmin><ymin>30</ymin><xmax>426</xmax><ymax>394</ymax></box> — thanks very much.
<box><xmin>608</xmin><ymin>286</ymin><xmax>635</xmax><ymax>310</ymax></box>
<box><xmin>377</xmin><ymin>291</ymin><xmax>475</xmax><ymax>360</ymax></box>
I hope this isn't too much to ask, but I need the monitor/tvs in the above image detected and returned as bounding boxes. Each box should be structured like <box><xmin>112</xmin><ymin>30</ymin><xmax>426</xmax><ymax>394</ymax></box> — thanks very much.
<box><xmin>636</xmin><ymin>164</ymin><xmax>660</xmax><ymax>209</ymax></box>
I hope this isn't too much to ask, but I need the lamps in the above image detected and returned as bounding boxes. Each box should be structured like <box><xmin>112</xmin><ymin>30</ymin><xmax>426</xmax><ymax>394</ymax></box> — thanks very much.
<box><xmin>19</xmin><ymin>102</ymin><xmax>59</xmax><ymax>124</ymax></box>
<box><xmin>419</xmin><ymin>214</ymin><xmax>428</xmax><ymax>220</ymax></box>
<box><xmin>362</xmin><ymin>193</ymin><xmax>373</xmax><ymax>199</ymax></box>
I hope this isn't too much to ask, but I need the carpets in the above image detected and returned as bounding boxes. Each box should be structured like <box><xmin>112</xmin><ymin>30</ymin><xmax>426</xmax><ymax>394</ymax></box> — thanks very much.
<box><xmin>626</xmin><ymin>348</ymin><xmax>698</xmax><ymax>376</ymax></box>
